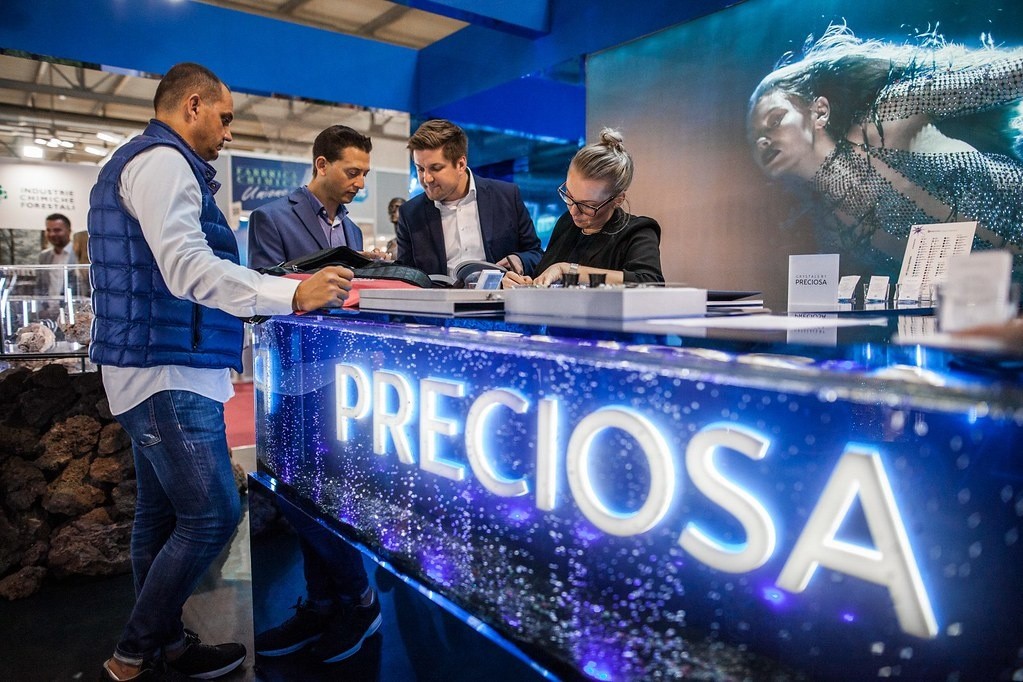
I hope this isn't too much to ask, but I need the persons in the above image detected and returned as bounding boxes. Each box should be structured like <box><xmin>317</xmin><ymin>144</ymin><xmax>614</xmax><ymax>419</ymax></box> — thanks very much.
<box><xmin>247</xmin><ymin>125</ymin><xmax>392</xmax><ymax>272</ymax></box>
<box><xmin>386</xmin><ymin>197</ymin><xmax>407</xmax><ymax>260</ymax></box>
<box><xmin>394</xmin><ymin>119</ymin><xmax>544</xmax><ymax>278</ymax></box>
<box><xmin>253</xmin><ymin>493</ymin><xmax>384</xmax><ymax>669</ymax></box>
<box><xmin>502</xmin><ymin>127</ymin><xmax>666</xmax><ymax>291</ymax></box>
<box><xmin>744</xmin><ymin>14</ymin><xmax>1023</xmax><ymax>292</ymax></box>
<box><xmin>35</xmin><ymin>215</ymin><xmax>80</xmax><ymax>320</ymax></box>
<box><xmin>85</xmin><ymin>60</ymin><xmax>352</xmax><ymax>682</ymax></box>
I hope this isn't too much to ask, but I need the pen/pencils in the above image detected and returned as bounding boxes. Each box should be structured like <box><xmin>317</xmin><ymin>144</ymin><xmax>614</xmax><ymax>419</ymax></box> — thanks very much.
<box><xmin>506</xmin><ymin>256</ymin><xmax>520</xmax><ymax>277</ymax></box>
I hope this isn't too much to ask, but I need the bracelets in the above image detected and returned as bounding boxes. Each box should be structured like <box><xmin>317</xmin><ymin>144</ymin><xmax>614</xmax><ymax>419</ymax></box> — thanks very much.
<box><xmin>570</xmin><ymin>262</ymin><xmax>580</xmax><ymax>275</ymax></box>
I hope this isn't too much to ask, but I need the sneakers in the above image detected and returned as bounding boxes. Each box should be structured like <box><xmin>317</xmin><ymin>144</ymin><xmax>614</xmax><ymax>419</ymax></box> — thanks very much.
<box><xmin>99</xmin><ymin>658</ymin><xmax>161</xmax><ymax>682</ymax></box>
<box><xmin>156</xmin><ymin>628</ymin><xmax>248</xmax><ymax>680</ymax></box>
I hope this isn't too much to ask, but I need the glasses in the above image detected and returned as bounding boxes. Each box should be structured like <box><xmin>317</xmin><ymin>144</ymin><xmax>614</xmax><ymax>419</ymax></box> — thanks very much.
<box><xmin>557</xmin><ymin>181</ymin><xmax>619</xmax><ymax>217</ymax></box>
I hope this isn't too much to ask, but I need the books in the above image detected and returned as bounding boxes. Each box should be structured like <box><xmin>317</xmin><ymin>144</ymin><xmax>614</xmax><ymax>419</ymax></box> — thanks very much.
<box><xmin>427</xmin><ymin>260</ymin><xmax>512</xmax><ymax>288</ymax></box>
<box><xmin>707</xmin><ymin>288</ymin><xmax>764</xmax><ymax>307</ymax></box>
